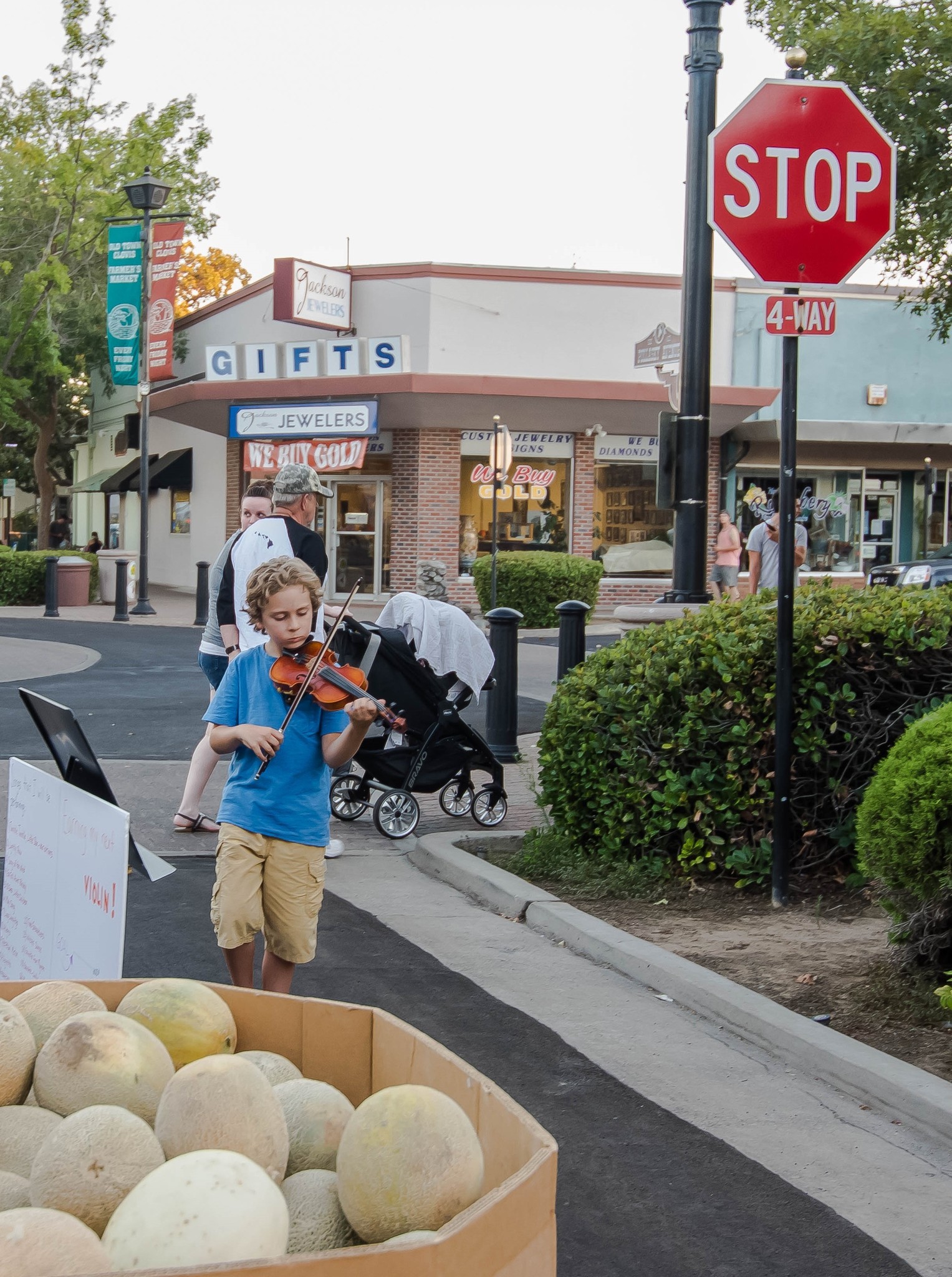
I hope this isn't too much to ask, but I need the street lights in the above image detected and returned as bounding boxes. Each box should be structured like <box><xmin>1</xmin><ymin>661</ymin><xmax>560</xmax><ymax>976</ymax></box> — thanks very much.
<box><xmin>104</xmin><ymin>164</ymin><xmax>193</xmax><ymax>616</ymax></box>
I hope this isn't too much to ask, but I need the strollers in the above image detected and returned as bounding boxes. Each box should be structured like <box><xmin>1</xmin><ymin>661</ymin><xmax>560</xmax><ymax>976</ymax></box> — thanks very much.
<box><xmin>320</xmin><ymin>613</ymin><xmax>509</xmax><ymax>839</ymax></box>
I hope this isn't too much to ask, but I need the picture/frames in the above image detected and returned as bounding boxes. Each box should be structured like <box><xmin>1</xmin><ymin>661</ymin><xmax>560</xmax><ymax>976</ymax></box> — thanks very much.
<box><xmin>602</xmin><ymin>488</ymin><xmax>674</xmax><ymax>546</ymax></box>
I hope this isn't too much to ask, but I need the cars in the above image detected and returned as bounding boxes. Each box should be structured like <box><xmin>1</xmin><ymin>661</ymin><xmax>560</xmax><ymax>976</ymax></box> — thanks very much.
<box><xmin>864</xmin><ymin>541</ymin><xmax>952</xmax><ymax>593</ymax></box>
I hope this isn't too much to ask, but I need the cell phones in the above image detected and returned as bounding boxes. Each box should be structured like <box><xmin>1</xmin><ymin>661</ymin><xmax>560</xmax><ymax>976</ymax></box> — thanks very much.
<box><xmin>765</xmin><ymin>523</ymin><xmax>776</xmax><ymax>531</ymax></box>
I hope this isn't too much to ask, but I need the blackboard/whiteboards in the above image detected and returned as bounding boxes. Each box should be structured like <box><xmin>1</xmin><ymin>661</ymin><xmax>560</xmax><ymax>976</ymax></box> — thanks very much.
<box><xmin>0</xmin><ymin>756</ymin><xmax>130</xmax><ymax>982</ymax></box>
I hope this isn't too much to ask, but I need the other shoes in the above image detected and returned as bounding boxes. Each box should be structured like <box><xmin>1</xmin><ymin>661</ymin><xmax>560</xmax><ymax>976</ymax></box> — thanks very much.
<box><xmin>324</xmin><ymin>839</ymin><xmax>345</xmax><ymax>858</ymax></box>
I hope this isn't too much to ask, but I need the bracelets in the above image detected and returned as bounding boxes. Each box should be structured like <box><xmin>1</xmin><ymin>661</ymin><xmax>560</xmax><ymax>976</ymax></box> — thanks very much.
<box><xmin>225</xmin><ymin>644</ymin><xmax>239</xmax><ymax>654</ymax></box>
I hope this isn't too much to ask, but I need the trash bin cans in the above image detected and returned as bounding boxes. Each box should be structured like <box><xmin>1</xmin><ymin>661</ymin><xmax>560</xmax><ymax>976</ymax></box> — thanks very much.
<box><xmin>56</xmin><ymin>556</ymin><xmax>92</xmax><ymax>607</ymax></box>
<box><xmin>97</xmin><ymin>549</ymin><xmax>139</xmax><ymax>604</ymax></box>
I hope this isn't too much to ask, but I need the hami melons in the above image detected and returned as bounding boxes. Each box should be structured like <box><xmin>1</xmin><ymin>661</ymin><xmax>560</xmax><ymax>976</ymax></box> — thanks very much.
<box><xmin>0</xmin><ymin>975</ymin><xmax>484</xmax><ymax>1277</ymax></box>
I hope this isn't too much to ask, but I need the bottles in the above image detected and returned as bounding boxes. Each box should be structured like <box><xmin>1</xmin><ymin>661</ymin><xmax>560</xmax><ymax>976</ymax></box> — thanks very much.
<box><xmin>458</xmin><ymin>514</ymin><xmax>479</xmax><ymax>568</ymax></box>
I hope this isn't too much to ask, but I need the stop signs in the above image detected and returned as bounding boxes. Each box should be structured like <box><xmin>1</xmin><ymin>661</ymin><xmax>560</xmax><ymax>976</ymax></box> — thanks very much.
<box><xmin>706</xmin><ymin>79</ymin><xmax>896</xmax><ymax>285</ymax></box>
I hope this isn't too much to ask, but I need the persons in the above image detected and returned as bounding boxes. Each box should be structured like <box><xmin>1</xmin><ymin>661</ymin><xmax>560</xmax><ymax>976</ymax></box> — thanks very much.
<box><xmin>49</xmin><ymin>515</ymin><xmax>69</xmax><ymax>548</ymax></box>
<box><xmin>83</xmin><ymin>532</ymin><xmax>104</xmax><ymax>553</ymax></box>
<box><xmin>203</xmin><ymin>556</ymin><xmax>386</xmax><ymax>994</ymax></box>
<box><xmin>746</xmin><ymin>490</ymin><xmax>808</xmax><ymax>595</ymax></box>
<box><xmin>218</xmin><ymin>463</ymin><xmax>353</xmax><ymax>858</ymax></box>
<box><xmin>710</xmin><ymin>509</ymin><xmax>742</xmax><ymax>602</ymax></box>
<box><xmin>173</xmin><ymin>479</ymin><xmax>274</xmax><ymax>832</ymax></box>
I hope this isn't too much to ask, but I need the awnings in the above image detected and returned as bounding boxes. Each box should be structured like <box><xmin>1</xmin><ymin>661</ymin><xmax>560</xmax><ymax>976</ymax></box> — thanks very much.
<box><xmin>120</xmin><ymin>448</ymin><xmax>192</xmax><ymax>490</ymax></box>
<box><xmin>65</xmin><ymin>454</ymin><xmax>158</xmax><ymax>495</ymax></box>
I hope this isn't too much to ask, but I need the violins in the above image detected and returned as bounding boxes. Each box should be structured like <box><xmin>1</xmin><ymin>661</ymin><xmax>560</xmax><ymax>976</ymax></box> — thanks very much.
<box><xmin>269</xmin><ymin>640</ymin><xmax>408</xmax><ymax>734</ymax></box>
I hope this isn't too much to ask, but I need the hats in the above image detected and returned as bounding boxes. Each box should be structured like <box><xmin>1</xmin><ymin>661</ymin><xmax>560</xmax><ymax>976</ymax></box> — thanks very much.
<box><xmin>273</xmin><ymin>463</ymin><xmax>334</xmax><ymax>498</ymax></box>
<box><xmin>719</xmin><ymin>510</ymin><xmax>731</xmax><ymax>516</ymax></box>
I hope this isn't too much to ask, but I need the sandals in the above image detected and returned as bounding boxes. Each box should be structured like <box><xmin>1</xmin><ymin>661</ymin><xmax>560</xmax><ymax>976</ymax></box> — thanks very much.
<box><xmin>173</xmin><ymin>811</ymin><xmax>221</xmax><ymax>832</ymax></box>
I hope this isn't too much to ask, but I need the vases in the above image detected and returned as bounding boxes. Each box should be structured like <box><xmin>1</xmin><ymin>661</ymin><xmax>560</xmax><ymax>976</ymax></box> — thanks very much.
<box><xmin>458</xmin><ymin>513</ymin><xmax>481</xmax><ymax>568</ymax></box>
